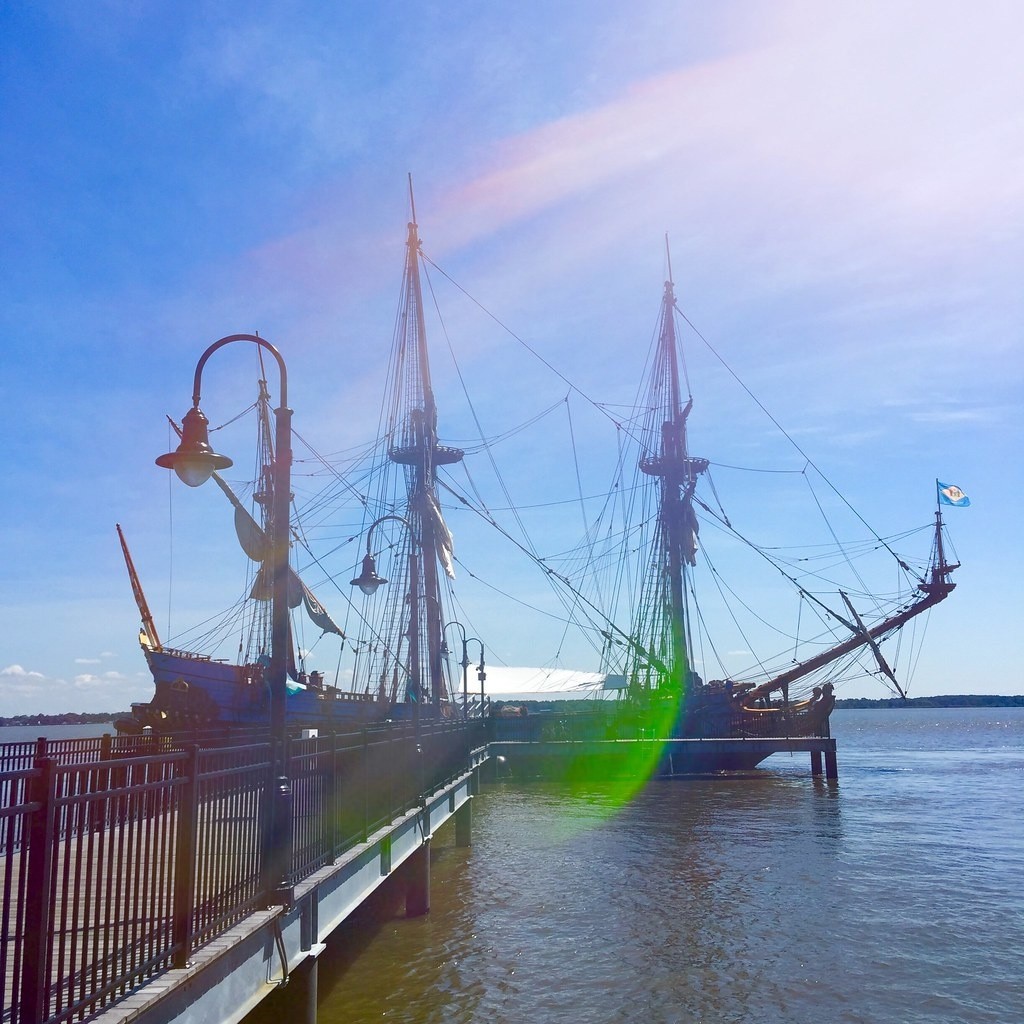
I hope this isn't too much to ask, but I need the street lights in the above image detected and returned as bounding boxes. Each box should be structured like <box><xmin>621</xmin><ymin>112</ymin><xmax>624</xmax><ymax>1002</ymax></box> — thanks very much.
<box><xmin>152</xmin><ymin>332</ymin><xmax>297</xmax><ymax>906</ymax></box>
<box><xmin>350</xmin><ymin>515</ymin><xmax>489</xmax><ymax>800</ymax></box>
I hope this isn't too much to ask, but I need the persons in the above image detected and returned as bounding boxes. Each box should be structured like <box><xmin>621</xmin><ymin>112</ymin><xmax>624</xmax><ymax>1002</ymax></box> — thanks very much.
<box><xmin>520</xmin><ymin>702</ymin><xmax>528</xmax><ymax>716</ymax></box>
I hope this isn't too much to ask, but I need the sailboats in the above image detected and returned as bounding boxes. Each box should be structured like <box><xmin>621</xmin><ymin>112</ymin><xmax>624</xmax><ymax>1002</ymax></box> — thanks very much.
<box><xmin>110</xmin><ymin>172</ymin><xmax>963</xmax><ymax>777</ymax></box>
